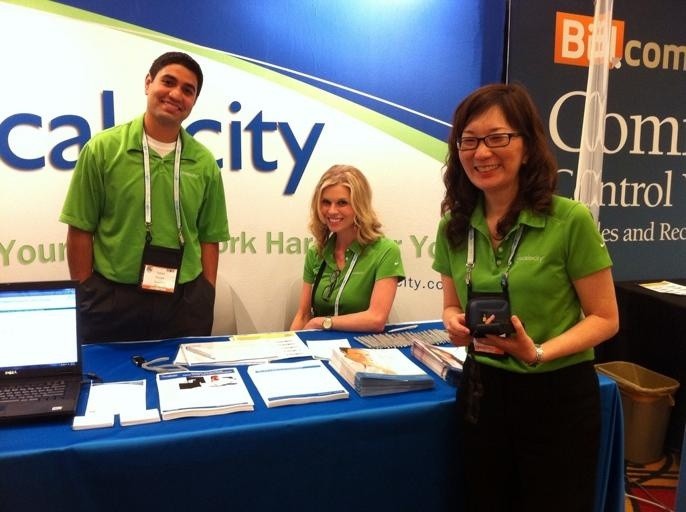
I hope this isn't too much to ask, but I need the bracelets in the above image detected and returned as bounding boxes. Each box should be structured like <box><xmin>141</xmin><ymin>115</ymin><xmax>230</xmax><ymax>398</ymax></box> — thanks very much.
<box><xmin>528</xmin><ymin>347</ymin><xmax>543</xmax><ymax>368</ymax></box>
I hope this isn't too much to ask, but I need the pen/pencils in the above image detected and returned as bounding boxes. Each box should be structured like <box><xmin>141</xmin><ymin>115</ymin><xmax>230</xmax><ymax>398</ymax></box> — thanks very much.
<box><xmin>402</xmin><ymin>329</ymin><xmax>452</xmax><ymax>347</ymax></box>
<box><xmin>388</xmin><ymin>324</ymin><xmax>419</xmax><ymax>333</ymax></box>
<box><xmin>353</xmin><ymin>333</ymin><xmax>402</xmax><ymax>349</ymax></box>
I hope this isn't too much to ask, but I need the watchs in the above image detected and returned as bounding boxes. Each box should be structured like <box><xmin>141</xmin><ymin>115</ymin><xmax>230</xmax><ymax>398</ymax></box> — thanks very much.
<box><xmin>322</xmin><ymin>315</ymin><xmax>333</xmax><ymax>331</ymax></box>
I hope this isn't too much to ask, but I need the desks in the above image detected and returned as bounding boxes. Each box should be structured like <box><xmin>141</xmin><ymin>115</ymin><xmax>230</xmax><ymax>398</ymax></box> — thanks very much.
<box><xmin>0</xmin><ymin>317</ymin><xmax>626</xmax><ymax>512</ymax></box>
<box><xmin>613</xmin><ymin>280</ymin><xmax>685</xmax><ymax>462</ymax></box>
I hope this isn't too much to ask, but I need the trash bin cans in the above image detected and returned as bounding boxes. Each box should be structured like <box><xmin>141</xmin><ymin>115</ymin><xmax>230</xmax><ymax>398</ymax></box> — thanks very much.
<box><xmin>594</xmin><ymin>360</ymin><xmax>680</xmax><ymax>465</ymax></box>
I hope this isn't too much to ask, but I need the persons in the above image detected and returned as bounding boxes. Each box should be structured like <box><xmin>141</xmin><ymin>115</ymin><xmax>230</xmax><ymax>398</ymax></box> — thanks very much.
<box><xmin>59</xmin><ymin>52</ymin><xmax>230</xmax><ymax>344</ymax></box>
<box><xmin>430</xmin><ymin>83</ymin><xmax>620</xmax><ymax>512</ymax></box>
<box><xmin>289</xmin><ymin>166</ymin><xmax>405</xmax><ymax>334</ymax></box>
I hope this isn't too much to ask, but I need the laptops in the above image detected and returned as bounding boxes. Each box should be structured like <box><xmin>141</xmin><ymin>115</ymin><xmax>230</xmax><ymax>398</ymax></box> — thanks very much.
<box><xmin>0</xmin><ymin>281</ymin><xmax>83</xmax><ymax>420</ymax></box>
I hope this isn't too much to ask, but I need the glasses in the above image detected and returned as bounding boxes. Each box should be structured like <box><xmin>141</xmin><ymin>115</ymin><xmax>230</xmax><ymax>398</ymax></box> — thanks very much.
<box><xmin>457</xmin><ymin>133</ymin><xmax>523</xmax><ymax>150</ymax></box>
<box><xmin>322</xmin><ymin>270</ymin><xmax>340</xmax><ymax>301</ymax></box>
